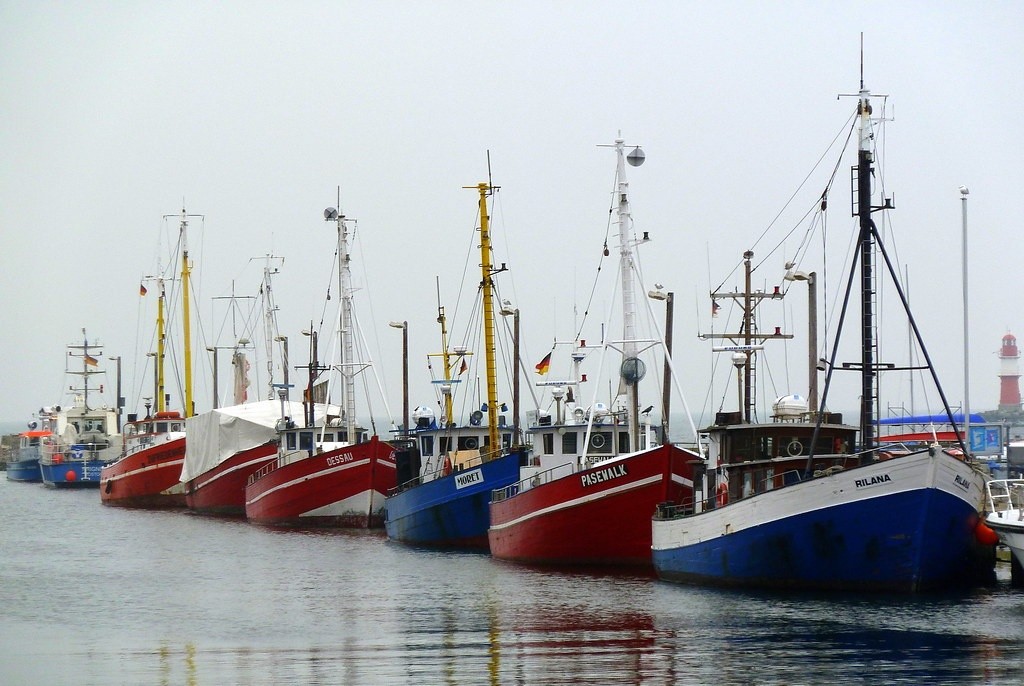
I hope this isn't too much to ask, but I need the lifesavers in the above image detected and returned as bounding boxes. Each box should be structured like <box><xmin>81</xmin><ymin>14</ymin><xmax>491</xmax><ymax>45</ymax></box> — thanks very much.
<box><xmin>715</xmin><ymin>482</ymin><xmax>728</xmax><ymax>507</ymax></box>
<box><xmin>157</xmin><ymin>411</ymin><xmax>180</xmax><ymax>418</ymax></box>
<box><xmin>444</xmin><ymin>458</ymin><xmax>451</xmax><ymax>475</ymax></box>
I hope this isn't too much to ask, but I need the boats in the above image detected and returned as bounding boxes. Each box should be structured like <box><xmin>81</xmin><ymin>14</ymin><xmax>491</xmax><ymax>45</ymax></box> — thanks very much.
<box><xmin>670</xmin><ymin>249</ymin><xmax>863</xmax><ymax>514</ymax></box>
<box><xmin>39</xmin><ymin>328</ymin><xmax>128</xmax><ymax>489</ymax></box>
<box><xmin>6</xmin><ymin>405</ymin><xmax>64</xmax><ymax>482</ymax></box>
<box><xmin>982</xmin><ymin>477</ymin><xmax>1024</xmax><ymax>586</ymax></box>
<box><xmin>853</xmin><ymin>399</ymin><xmax>1024</xmax><ymax>475</ymax></box>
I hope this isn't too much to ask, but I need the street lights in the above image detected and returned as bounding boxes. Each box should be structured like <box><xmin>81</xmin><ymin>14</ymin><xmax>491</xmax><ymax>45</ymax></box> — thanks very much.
<box><xmin>301</xmin><ymin>330</ymin><xmax>319</xmax><ymax>384</ymax></box>
<box><xmin>647</xmin><ymin>288</ymin><xmax>674</xmax><ymax>444</ymax></box>
<box><xmin>203</xmin><ymin>345</ymin><xmax>219</xmax><ymax>410</ymax></box>
<box><xmin>958</xmin><ymin>183</ymin><xmax>974</xmax><ymax>455</ymax></box>
<box><xmin>109</xmin><ymin>355</ymin><xmax>122</xmax><ymax>433</ymax></box>
<box><xmin>387</xmin><ymin>321</ymin><xmax>410</xmax><ymax>436</ymax></box>
<box><xmin>274</xmin><ymin>335</ymin><xmax>291</xmax><ymax>401</ymax></box>
<box><xmin>498</xmin><ymin>306</ymin><xmax>521</xmax><ymax>453</ymax></box>
<box><xmin>785</xmin><ymin>267</ymin><xmax>822</xmax><ymax>420</ymax></box>
<box><xmin>145</xmin><ymin>353</ymin><xmax>159</xmax><ymax>414</ymax></box>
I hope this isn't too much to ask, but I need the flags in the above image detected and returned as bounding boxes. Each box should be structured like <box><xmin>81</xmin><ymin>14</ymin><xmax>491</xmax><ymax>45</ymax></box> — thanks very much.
<box><xmin>140</xmin><ymin>284</ymin><xmax>147</xmax><ymax>296</ymax></box>
<box><xmin>85</xmin><ymin>354</ymin><xmax>98</xmax><ymax>366</ymax></box>
<box><xmin>535</xmin><ymin>352</ymin><xmax>552</xmax><ymax>375</ymax></box>
<box><xmin>712</xmin><ymin>299</ymin><xmax>720</xmax><ymax>317</ymax></box>
<box><xmin>458</xmin><ymin>358</ymin><xmax>467</xmax><ymax>375</ymax></box>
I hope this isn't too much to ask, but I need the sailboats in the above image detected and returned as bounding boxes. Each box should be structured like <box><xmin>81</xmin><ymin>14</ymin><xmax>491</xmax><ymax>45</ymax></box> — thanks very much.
<box><xmin>242</xmin><ymin>186</ymin><xmax>412</xmax><ymax>531</ymax></box>
<box><xmin>183</xmin><ymin>252</ymin><xmax>347</xmax><ymax>516</ymax></box>
<box><xmin>381</xmin><ymin>151</ymin><xmax>528</xmax><ymax>550</ymax></box>
<box><xmin>649</xmin><ymin>35</ymin><xmax>996</xmax><ymax>599</ymax></box>
<box><xmin>486</xmin><ymin>131</ymin><xmax>709</xmax><ymax>568</ymax></box>
<box><xmin>99</xmin><ymin>194</ymin><xmax>206</xmax><ymax>510</ymax></box>
